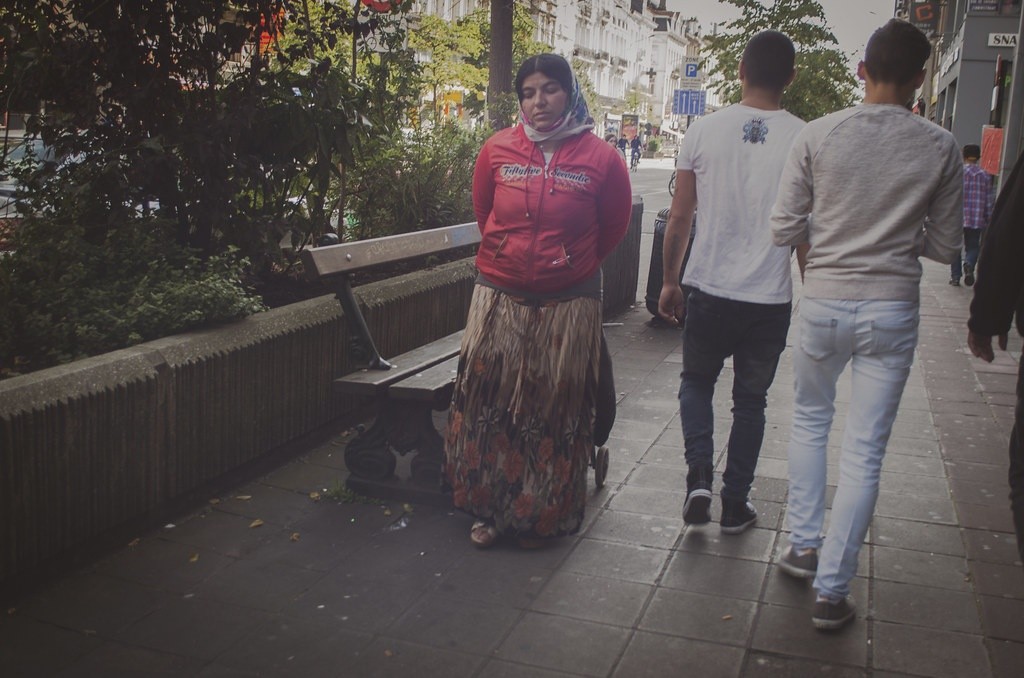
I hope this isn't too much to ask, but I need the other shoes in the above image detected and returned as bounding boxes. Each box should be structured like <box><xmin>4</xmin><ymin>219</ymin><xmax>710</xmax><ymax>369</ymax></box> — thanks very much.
<box><xmin>963</xmin><ymin>261</ymin><xmax>974</xmax><ymax>286</ymax></box>
<box><xmin>949</xmin><ymin>278</ymin><xmax>960</xmax><ymax>286</ymax></box>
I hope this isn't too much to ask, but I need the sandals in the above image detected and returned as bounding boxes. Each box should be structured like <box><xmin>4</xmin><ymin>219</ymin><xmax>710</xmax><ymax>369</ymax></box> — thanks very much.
<box><xmin>521</xmin><ymin>537</ymin><xmax>543</xmax><ymax>548</ymax></box>
<box><xmin>471</xmin><ymin>521</ymin><xmax>499</xmax><ymax>546</ymax></box>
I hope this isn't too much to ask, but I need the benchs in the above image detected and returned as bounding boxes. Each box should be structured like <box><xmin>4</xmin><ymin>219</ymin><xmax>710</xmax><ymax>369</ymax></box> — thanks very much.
<box><xmin>306</xmin><ymin>221</ymin><xmax>481</xmax><ymax>504</ymax></box>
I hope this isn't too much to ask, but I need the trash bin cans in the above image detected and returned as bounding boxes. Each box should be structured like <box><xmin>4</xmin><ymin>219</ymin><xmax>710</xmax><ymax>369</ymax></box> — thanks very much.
<box><xmin>600</xmin><ymin>201</ymin><xmax>644</xmax><ymax>325</ymax></box>
<box><xmin>644</xmin><ymin>208</ymin><xmax>697</xmax><ymax>322</ymax></box>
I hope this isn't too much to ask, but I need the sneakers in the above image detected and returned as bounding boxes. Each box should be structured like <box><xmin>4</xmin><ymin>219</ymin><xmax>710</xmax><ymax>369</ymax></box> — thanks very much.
<box><xmin>812</xmin><ymin>594</ymin><xmax>855</xmax><ymax>629</ymax></box>
<box><xmin>682</xmin><ymin>481</ymin><xmax>712</xmax><ymax>524</ymax></box>
<box><xmin>720</xmin><ymin>502</ymin><xmax>757</xmax><ymax>533</ymax></box>
<box><xmin>778</xmin><ymin>545</ymin><xmax>818</xmax><ymax>578</ymax></box>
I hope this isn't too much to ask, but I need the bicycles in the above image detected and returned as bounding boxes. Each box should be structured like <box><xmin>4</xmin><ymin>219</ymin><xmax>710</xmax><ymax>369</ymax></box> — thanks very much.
<box><xmin>633</xmin><ymin>153</ymin><xmax>639</xmax><ymax>171</ymax></box>
<box><xmin>669</xmin><ymin>171</ymin><xmax>677</xmax><ymax>198</ymax></box>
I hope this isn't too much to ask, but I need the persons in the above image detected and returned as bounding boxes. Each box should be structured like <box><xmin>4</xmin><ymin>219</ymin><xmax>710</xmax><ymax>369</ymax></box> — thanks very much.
<box><xmin>950</xmin><ymin>145</ymin><xmax>995</xmax><ymax>285</ymax></box>
<box><xmin>658</xmin><ymin>31</ymin><xmax>806</xmax><ymax>534</ymax></box>
<box><xmin>444</xmin><ymin>54</ymin><xmax>632</xmax><ymax>547</ymax></box>
<box><xmin>770</xmin><ymin>21</ymin><xmax>962</xmax><ymax>630</ymax></box>
<box><xmin>967</xmin><ymin>152</ymin><xmax>1024</xmax><ymax>561</ymax></box>
<box><xmin>605</xmin><ymin>133</ymin><xmax>643</xmax><ymax>169</ymax></box>
